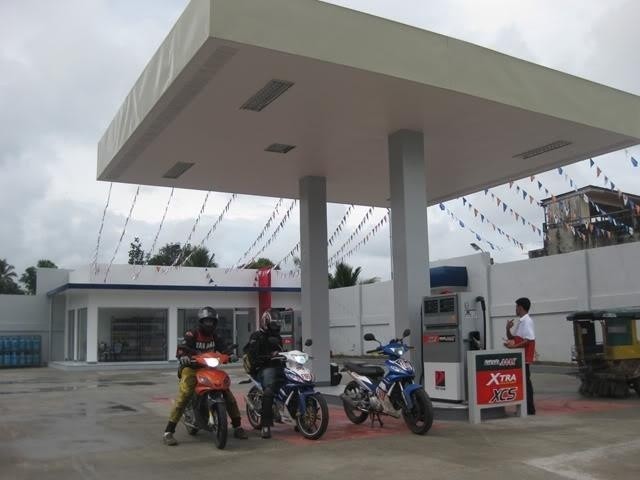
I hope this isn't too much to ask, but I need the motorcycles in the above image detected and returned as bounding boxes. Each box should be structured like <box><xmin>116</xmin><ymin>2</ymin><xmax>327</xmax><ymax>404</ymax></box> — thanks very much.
<box><xmin>338</xmin><ymin>329</ymin><xmax>433</xmax><ymax>435</ymax></box>
<box><xmin>175</xmin><ymin>330</ymin><xmax>243</xmax><ymax>449</ymax></box>
<box><xmin>237</xmin><ymin>337</ymin><xmax>329</xmax><ymax>440</ymax></box>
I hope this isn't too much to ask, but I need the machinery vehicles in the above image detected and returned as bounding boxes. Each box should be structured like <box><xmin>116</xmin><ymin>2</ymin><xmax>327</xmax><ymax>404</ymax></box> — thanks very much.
<box><xmin>564</xmin><ymin>304</ymin><xmax>639</xmax><ymax>399</ymax></box>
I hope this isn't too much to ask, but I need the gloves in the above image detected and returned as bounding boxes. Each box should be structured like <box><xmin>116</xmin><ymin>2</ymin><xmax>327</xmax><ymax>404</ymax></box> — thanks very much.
<box><xmin>180</xmin><ymin>355</ymin><xmax>192</xmax><ymax>367</ymax></box>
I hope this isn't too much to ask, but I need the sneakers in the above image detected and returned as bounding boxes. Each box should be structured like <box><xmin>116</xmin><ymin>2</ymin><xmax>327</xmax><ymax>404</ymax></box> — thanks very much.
<box><xmin>163</xmin><ymin>431</ymin><xmax>177</xmax><ymax>446</ymax></box>
<box><xmin>260</xmin><ymin>424</ymin><xmax>271</xmax><ymax>438</ymax></box>
<box><xmin>234</xmin><ymin>427</ymin><xmax>247</xmax><ymax>439</ymax></box>
<box><xmin>295</xmin><ymin>424</ymin><xmax>312</xmax><ymax>433</ymax></box>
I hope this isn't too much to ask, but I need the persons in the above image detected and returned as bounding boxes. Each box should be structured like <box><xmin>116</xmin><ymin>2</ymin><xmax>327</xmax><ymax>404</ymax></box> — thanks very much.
<box><xmin>501</xmin><ymin>296</ymin><xmax>538</xmax><ymax>416</ymax></box>
<box><xmin>245</xmin><ymin>306</ymin><xmax>313</xmax><ymax>441</ymax></box>
<box><xmin>161</xmin><ymin>306</ymin><xmax>250</xmax><ymax>447</ymax></box>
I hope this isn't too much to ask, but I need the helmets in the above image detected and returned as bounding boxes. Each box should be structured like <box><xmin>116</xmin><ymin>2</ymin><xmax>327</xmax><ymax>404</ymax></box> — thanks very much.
<box><xmin>261</xmin><ymin>308</ymin><xmax>282</xmax><ymax>336</ymax></box>
<box><xmin>198</xmin><ymin>306</ymin><xmax>218</xmax><ymax>336</ymax></box>
